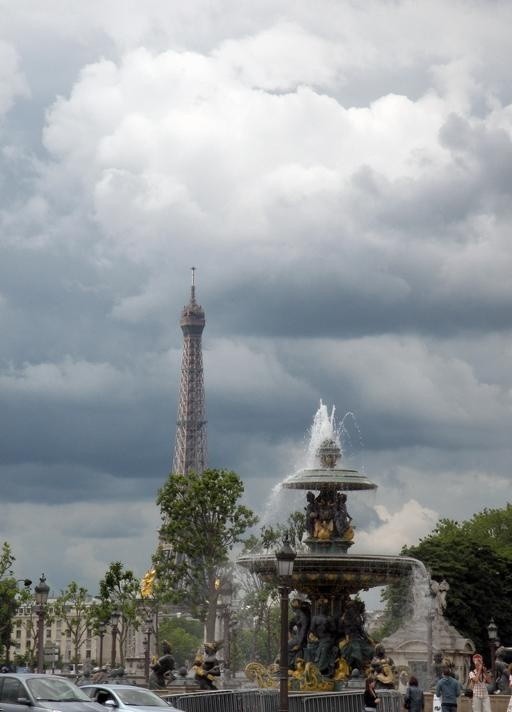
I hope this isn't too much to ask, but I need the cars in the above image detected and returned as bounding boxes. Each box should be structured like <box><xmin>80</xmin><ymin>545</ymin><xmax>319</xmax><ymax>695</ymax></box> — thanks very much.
<box><xmin>60</xmin><ymin>683</ymin><xmax>186</xmax><ymax>712</ymax></box>
<box><xmin>0</xmin><ymin>672</ymin><xmax>110</xmax><ymax>711</ymax></box>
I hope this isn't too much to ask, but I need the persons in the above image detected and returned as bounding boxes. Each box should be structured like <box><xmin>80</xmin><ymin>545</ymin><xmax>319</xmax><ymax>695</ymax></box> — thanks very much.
<box><xmin>495</xmin><ymin>646</ymin><xmax>512</xmax><ymax>695</ymax></box>
<box><xmin>405</xmin><ymin>676</ymin><xmax>425</xmax><ymax>712</ymax></box>
<box><xmin>276</xmin><ymin>599</ymin><xmax>374</xmax><ymax>678</ymax></box>
<box><xmin>469</xmin><ymin>661</ymin><xmax>492</xmax><ymax>712</ymax></box>
<box><xmin>194</xmin><ymin>641</ymin><xmax>220</xmax><ymax>691</ymax></box>
<box><xmin>149</xmin><ymin>640</ymin><xmax>175</xmax><ymax>690</ymax></box>
<box><xmin>436</xmin><ymin>669</ymin><xmax>461</xmax><ymax>712</ymax></box>
<box><xmin>304</xmin><ymin>491</ymin><xmax>353</xmax><ymax>537</ymax></box>
<box><xmin>371</xmin><ymin>644</ymin><xmax>397</xmax><ymax>689</ymax></box>
<box><xmin>432</xmin><ymin>653</ymin><xmax>446</xmax><ymax>683</ymax></box>
<box><xmin>364</xmin><ymin>678</ymin><xmax>381</xmax><ymax>712</ymax></box>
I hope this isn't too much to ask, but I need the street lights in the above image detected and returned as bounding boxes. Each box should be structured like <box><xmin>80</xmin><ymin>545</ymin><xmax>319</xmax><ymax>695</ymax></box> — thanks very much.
<box><xmin>274</xmin><ymin>529</ymin><xmax>296</xmax><ymax>712</ymax></box>
<box><xmin>145</xmin><ymin>614</ymin><xmax>153</xmax><ymax>678</ymax></box>
<box><xmin>110</xmin><ymin>612</ymin><xmax>120</xmax><ymax>669</ymax></box>
<box><xmin>33</xmin><ymin>572</ymin><xmax>50</xmax><ymax>673</ymax></box>
<box><xmin>486</xmin><ymin>616</ymin><xmax>499</xmax><ymax>695</ymax></box>
<box><xmin>98</xmin><ymin>622</ymin><xmax>105</xmax><ymax>669</ymax></box>
<box><xmin>142</xmin><ymin>637</ymin><xmax>148</xmax><ymax>676</ymax></box>
